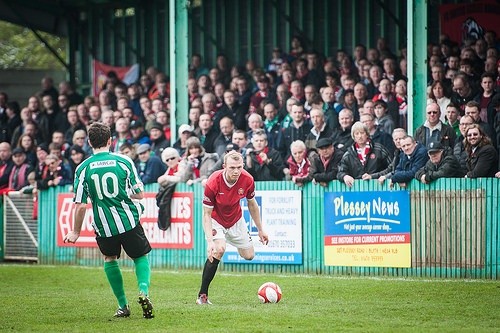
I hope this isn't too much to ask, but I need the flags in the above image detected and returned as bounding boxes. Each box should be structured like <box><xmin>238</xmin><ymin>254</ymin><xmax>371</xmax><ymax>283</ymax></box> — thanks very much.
<box><xmin>439</xmin><ymin>0</ymin><xmax>500</xmax><ymax>43</ymax></box>
<box><xmin>93</xmin><ymin>59</ymin><xmax>139</xmax><ymax>95</ymax></box>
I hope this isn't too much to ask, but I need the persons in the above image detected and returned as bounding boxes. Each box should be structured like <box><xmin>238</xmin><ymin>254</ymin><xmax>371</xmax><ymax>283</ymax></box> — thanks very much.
<box><xmin>308</xmin><ymin>137</ymin><xmax>344</xmax><ymax>185</ymax></box>
<box><xmin>414</xmin><ymin>141</ymin><xmax>461</xmax><ymax>184</ymax></box>
<box><xmin>244</xmin><ymin>129</ymin><xmax>285</xmax><ymax>181</ymax></box>
<box><xmin>63</xmin><ymin>121</ymin><xmax>154</xmax><ymax>319</ymax></box>
<box><xmin>463</xmin><ymin>125</ymin><xmax>497</xmax><ymax>179</ymax></box>
<box><xmin>196</xmin><ymin>150</ymin><xmax>269</xmax><ymax>305</ymax></box>
<box><xmin>389</xmin><ymin>135</ymin><xmax>431</xmax><ymax>188</ymax></box>
<box><xmin>0</xmin><ymin>30</ymin><xmax>500</xmax><ymax>192</ymax></box>
<box><xmin>336</xmin><ymin>123</ymin><xmax>392</xmax><ymax>187</ymax></box>
<box><xmin>286</xmin><ymin>139</ymin><xmax>317</xmax><ymax>186</ymax></box>
<box><xmin>364</xmin><ymin>128</ymin><xmax>407</xmax><ymax>184</ymax></box>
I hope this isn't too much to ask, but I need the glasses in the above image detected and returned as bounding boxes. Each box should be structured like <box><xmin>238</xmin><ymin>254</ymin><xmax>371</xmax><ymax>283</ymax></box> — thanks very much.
<box><xmin>427</xmin><ymin>111</ymin><xmax>437</xmax><ymax>115</ymax></box>
<box><xmin>166</xmin><ymin>157</ymin><xmax>176</xmax><ymax>162</ymax></box>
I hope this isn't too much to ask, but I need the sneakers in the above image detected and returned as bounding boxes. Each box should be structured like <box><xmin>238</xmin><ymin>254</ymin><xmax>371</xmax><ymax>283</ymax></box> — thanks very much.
<box><xmin>138</xmin><ymin>295</ymin><xmax>154</xmax><ymax>318</ymax></box>
<box><xmin>114</xmin><ymin>304</ymin><xmax>131</xmax><ymax>318</ymax></box>
<box><xmin>195</xmin><ymin>292</ymin><xmax>212</xmax><ymax>306</ymax></box>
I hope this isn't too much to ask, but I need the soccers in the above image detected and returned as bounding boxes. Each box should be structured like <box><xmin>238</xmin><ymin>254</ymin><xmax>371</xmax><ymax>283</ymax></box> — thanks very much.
<box><xmin>258</xmin><ymin>282</ymin><xmax>282</xmax><ymax>304</ymax></box>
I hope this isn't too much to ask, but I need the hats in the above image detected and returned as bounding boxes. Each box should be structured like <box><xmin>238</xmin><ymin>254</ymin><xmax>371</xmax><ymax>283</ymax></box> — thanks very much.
<box><xmin>315</xmin><ymin>138</ymin><xmax>333</xmax><ymax>150</ymax></box>
<box><xmin>146</xmin><ymin>122</ymin><xmax>165</xmax><ymax>132</ymax></box>
<box><xmin>178</xmin><ymin>124</ymin><xmax>193</xmax><ymax>134</ymax></box>
<box><xmin>128</xmin><ymin>120</ymin><xmax>142</xmax><ymax>129</ymax></box>
<box><xmin>9</xmin><ymin>147</ymin><xmax>25</xmax><ymax>155</ymax></box>
<box><xmin>184</xmin><ymin>136</ymin><xmax>205</xmax><ymax>158</ymax></box>
<box><xmin>257</xmin><ymin>74</ymin><xmax>269</xmax><ymax>82</ymax></box>
<box><xmin>427</xmin><ymin>141</ymin><xmax>445</xmax><ymax>152</ymax></box>
<box><xmin>160</xmin><ymin>146</ymin><xmax>180</xmax><ymax>162</ymax></box>
<box><xmin>351</xmin><ymin>121</ymin><xmax>370</xmax><ymax>140</ymax></box>
<box><xmin>136</xmin><ymin>144</ymin><xmax>150</xmax><ymax>155</ymax></box>
